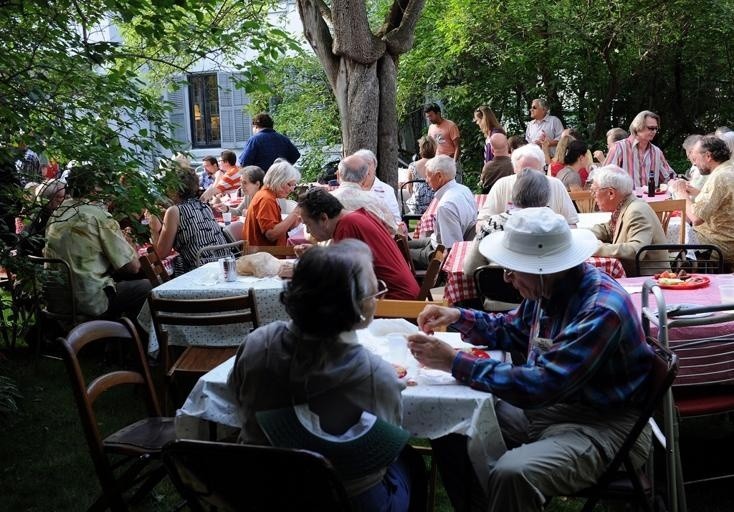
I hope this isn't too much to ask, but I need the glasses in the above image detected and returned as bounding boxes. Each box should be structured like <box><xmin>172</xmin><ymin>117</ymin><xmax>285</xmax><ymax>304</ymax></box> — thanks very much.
<box><xmin>356</xmin><ymin>279</ymin><xmax>388</xmax><ymax>309</ymax></box>
<box><xmin>501</xmin><ymin>268</ymin><xmax>517</xmax><ymax>281</ymax></box>
<box><xmin>646</xmin><ymin>124</ymin><xmax>659</xmax><ymax>131</ymax></box>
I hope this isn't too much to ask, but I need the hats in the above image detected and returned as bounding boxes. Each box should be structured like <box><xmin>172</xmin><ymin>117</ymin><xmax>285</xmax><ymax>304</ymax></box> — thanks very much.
<box><xmin>477</xmin><ymin>206</ymin><xmax>603</xmax><ymax>276</ymax></box>
<box><xmin>256</xmin><ymin>404</ymin><xmax>413</xmax><ymax>480</ymax></box>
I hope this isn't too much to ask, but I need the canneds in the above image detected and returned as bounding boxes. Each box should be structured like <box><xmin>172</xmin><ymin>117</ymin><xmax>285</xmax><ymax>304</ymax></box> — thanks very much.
<box><xmin>223</xmin><ymin>257</ymin><xmax>237</xmax><ymax>282</ymax></box>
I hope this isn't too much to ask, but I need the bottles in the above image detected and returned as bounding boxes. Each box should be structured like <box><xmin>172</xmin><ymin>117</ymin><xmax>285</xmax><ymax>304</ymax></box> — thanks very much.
<box><xmin>648</xmin><ymin>169</ymin><xmax>656</xmax><ymax>197</ymax></box>
<box><xmin>668</xmin><ymin>172</ymin><xmax>676</xmax><ymax>198</ymax></box>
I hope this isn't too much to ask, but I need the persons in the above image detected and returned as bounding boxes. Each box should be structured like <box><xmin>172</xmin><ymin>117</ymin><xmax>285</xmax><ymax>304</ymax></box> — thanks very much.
<box><xmin>407</xmin><ymin>103</ymin><xmax>476</xmax><ymax>270</ymax></box>
<box><xmin>227</xmin><ymin>240</ymin><xmax>428</xmax><ymax>511</ymax></box>
<box><xmin>409</xmin><ymin>207</ymin><xmax>652</xmax><ymax>511</ymax></box>
<box><xmin>201</xmin><ymin>150</ymin><xmax>423</xmax><ymax>324</ymax></box>
<box><xmin>238</xmin><ymin>114</ymin><xmax>300</xmax><ymax>171</ymax></box>
<box><xmin>13</xmin><ymin>133</ymin><xmax>231</xmax><ymax>375</ymax></box>
<box><xmin>474</xmin><ymin>99</ymin><xmax>733</xmax><ymax>311</ymax></box>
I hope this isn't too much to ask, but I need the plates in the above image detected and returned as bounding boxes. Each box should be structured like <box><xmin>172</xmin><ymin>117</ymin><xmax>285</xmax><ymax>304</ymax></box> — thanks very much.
<box><xmin>655</xmin><ymin>275</ymin><xmax>710</xmax><ymax>289</ymax></box>
<box><xmin>658</xmin><ymin>302</ymin><xmax>714</xmax><ymax>319</ymax></box>
<box><xmin>643</xmin><ymin>188</ymin><xmax>665</xmax><ymax>194</ymax></box>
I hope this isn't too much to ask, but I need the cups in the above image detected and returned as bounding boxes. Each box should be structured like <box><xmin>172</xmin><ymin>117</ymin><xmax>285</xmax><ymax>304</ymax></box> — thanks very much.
<box><xmin>385</xmin><ymin>330</ymin><xmax>409</xmax><ymax>364</ymax></box>
<box><xmin>221</xmin><ymin>212</ymin><xmax>231</xmax><ymax>225</ymax></box>
<box><xmin>230</xmin><ymin>192</ymin><xmax>237</xmax><ymax>201</ymax></box>
<box><xmin>716</xmin><ymin>273</ymin><xmax>734</xmax><ymax>312</ymax></box>
